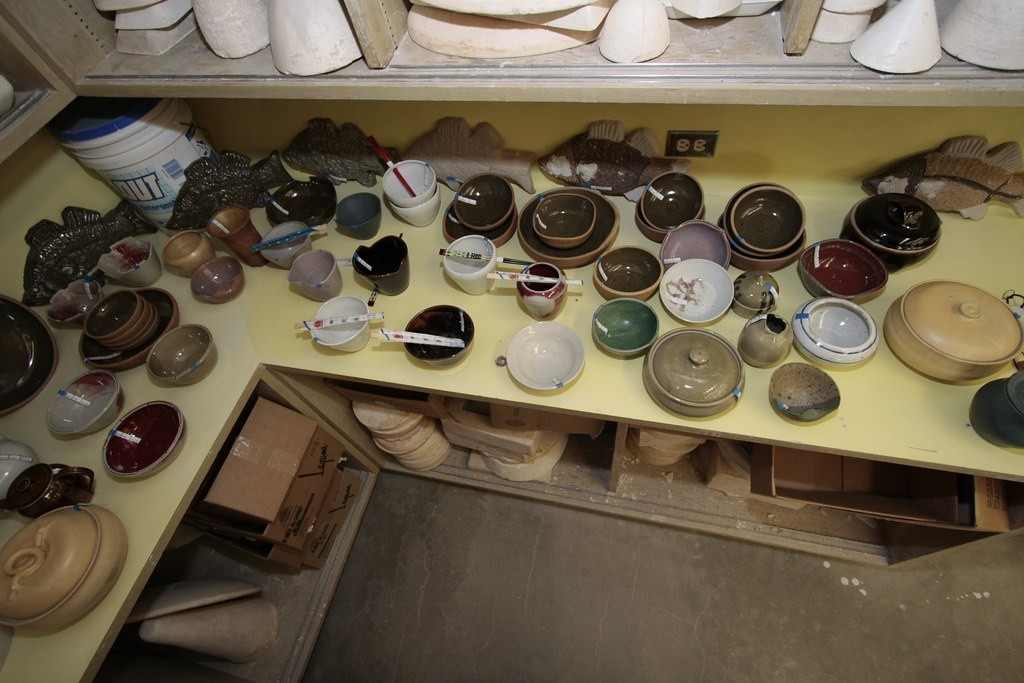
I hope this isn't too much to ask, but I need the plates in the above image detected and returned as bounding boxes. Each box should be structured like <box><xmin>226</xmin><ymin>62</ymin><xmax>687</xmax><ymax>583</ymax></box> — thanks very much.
<box><xmin>102</xmin><ymin>400</ymin><xmax>186</xmax><ymax>478</ymax></box>
<box><xmin>660</xmin><ymin>220</ymin><xmax>733</xmax><ymax>271</ymax></box>
<box><xmin>516</xmin><ymin>187</ymin><xmax>621</xmax><ymax>268</ymax></box>
<box><xmin>404</xmin><ymin>304</ymin><xmax>475</xmax><ymax>366</ymax></box>
<box><xmin>443</xmin><ymin>201</ymin><xmax>518</xmax><ymax>249</ymax></box>
<box><xmin>660</xmin><ymin>259</ymin><xmax>735</xmax><ymax>324</ymax></box>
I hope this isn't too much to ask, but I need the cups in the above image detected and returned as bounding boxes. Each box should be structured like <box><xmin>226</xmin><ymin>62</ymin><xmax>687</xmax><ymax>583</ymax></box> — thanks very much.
<box><xmin>389</xmin><ymin>185</ymin><xmax>441</xmax><ymax>227</ymax></box>
<box><xmin>208</xmin><ymin>207</ymin><xmax>268</xmax><ymax>267</ymax></box>
<box><xmin>163</xmin><ymin>231</ymin><xmax>216</xmax><ymax>278</ymax></box>
<box><xmin>47</xmin><ymin>279</ymin><xmax>104</xmax><ymax>326</ymax></box>
<box><xmin>260</xmin><ymin>222</ymin><xmax>312</xmax><ymax>269</ymax></box>
<box><xmin>311</xmin><ymin>296</ymin><xmax>370</xmax><ymax>352</ymax></box>
<box><xmin>335</xmin><ymin>193</ymin><xmax>381</xmax><ymax>238</ymax></box>
<box><xmin>442</xmin><ymin>235</ymin><xmax>497</xmax><ymax>295</ymax></box>
<box><xmin>731</xmin><ymin>271</ymin><xmax>779</xmax><ymax>319</ymax></box>
<box><xmin>517</xmin><ymin>262</ymin><xmax>568</xmax><ymax>321</ymax></box>
<box><xmin>287</xmin><ymin>250</ymin><xmax>343</xmax><ymax>301</ymax></box>
<box><xmin>969</xmin><ymin>371</ymin><xmax>1024</xmax><ymax>450</ymax></box>
<box><xmin>0</xmin><ymin>462</ymin><xmax>94</xmax><ymax>520</ymax></box>
<box><xmin>98</xmin><ymin>237</ymin><xmax>161</xmax><ymax>287</ymax></box>
<box><xmin>352</xmin><ymin>235</ymin><xmax>410</xmax><ymax>296</ymax></box>
<box><xmin>381</xmin><ymin>160</ymin><xmax>437</xmax><ymax>207</ymax></box>
<box><xmin>507</xmin><ymin>321</ymin><xmax>585</xmax><ymax>391</ymax></box>
<box><xmin>738</xmin><ymin>313</ymin><xmax>793</xmax><ymax>369</ymax></box>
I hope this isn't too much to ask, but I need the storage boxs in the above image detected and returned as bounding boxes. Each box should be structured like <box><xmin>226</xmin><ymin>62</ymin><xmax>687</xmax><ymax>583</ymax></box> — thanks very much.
<box><xmin>302</xmin><ymin>464</ymin><xmax>363</xmax><ymax>569</ymax></box>
<box><xmin>489</xmin><ymin>401</ymin><xmax>606</xmax><ymax>438</ymax></box>
<box><xmin>181</xmin><ymin>425</ymin><xmax>344</xmax><ymax>550</ymax></box>
<box><xmin>190</xmin><ymin>394</ymin><xmax>318</xmax><ymax>523</ymax></box>
<box><xmin>823</xmin><ymin>474</ymin><xmax>1011</xmax><ymax>533</ymax></box>
<box><xmin>326</xmin><ymin>376</ymin><xmax>449</xmax><ymax>418</ymax></box>
<box><xmin>751</xmin><ymin>442</ymin><xmax>958</xmax><ymax>525</ymax></box>
<box><xmin>185</xmin><ymin>466</ymin><xmax>344</xmax><ymax>570</ymax></box>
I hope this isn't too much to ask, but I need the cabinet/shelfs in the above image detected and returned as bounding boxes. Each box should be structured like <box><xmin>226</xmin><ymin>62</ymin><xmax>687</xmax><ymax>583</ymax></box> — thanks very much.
<box><xmin>0</xmin><ymin>0</ymin><xmax>1023</xmax><ymax>683</ymax></box>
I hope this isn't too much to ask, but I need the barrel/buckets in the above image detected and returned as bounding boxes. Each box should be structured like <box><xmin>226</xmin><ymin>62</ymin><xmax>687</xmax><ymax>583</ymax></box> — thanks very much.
<box><xmin>50</xmin><ymin>94</ymin><xmax>220</xmax><ymax>237</ymax></box>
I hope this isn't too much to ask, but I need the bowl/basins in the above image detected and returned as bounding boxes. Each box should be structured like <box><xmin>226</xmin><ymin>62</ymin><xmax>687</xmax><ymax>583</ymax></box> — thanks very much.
<box><xmin>47</xmin><ymin>370</ymin><xmax>124</xmax><ymax>435</ymax></box>
<box><xmin>532</xmin><ymin>194</ymin><xmax>597</xmax><ymax>250</ymax></box>
<box><xmin>771</xmin><ymin>363</ymin><xmax>841</xmax><ymax>421</ymax></box>
<box><xmin>717</xmin><ymin>181</ymin><xmax>806</xmax><ymax>272</ymax></box>
<box><xmin>641</xmin><ymin>171</ymin><xmax>705</xmax><ymax>232</ymax></box>
<box><xmin>191</xmin><ymin>257</ymin><xmax>245</xmax><ymax>304</ymax></box>
<box><xmin>792</xmin><ymin>296</ymin><xmax>879</xmax><ymax>367</ymax></box>
<box><xmin>839</xmin><ymin>192</ymin><xmax>941</xmax><ymax>273</ymax></box>
<box><xmin>591</xmin><ymin>298</ymin><xmax>660</xmax><ymax>356</ymax></box>
<box><xmin>79</xmin><ymin>287</ymin><xmax>179</xmax><ymax>372</ymax></box>
<box><xmin>147</xmin><ymin>324</ymin><xmax>218</xmax><ymax>384</ymax></box>
<box><xmin>634</xmin><ymin>196</ymin><xmax>706</xmax><ymax>243</ymax></box>
<box><xmin>885</xmin><ymin>280</ymin><xmax>1024</xmax><ymax>380</ymax></box>
<box><xmin>0</xmin><ymin>293</ymin><xmax>59</xmax><ymax>417</ymax></box>
<box><xmin>593</xmin><ymin>246</ymin><xmax>663</xmax><ymax>301</ymax></box>
<box><xmin>0</xmin><ymin>505</ymin><xmax>125</xmax><ymax>632</ymax></box>
<box><xmin>798</xmin><ymin>238</ymin><xmax>889</xmax><ymax>307</ymax></box>
<box><xmin>453</xmin><ymin>173</ymin><xmax>515</xmax><ymax>230</ymax></box>
